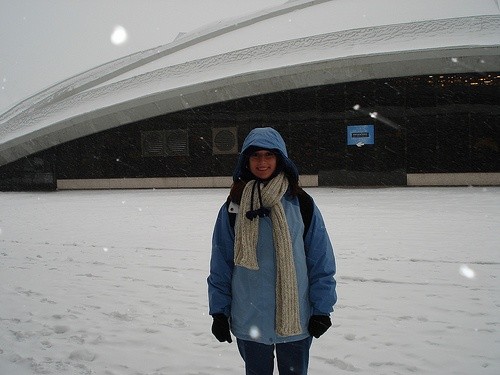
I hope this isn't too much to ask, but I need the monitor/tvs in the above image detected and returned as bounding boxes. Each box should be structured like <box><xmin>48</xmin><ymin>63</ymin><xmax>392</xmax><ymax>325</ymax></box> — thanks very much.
<box><xmin>347</xmin><ymin>125</ymin><xmax>374</xmax><ymax>145</ymax></box>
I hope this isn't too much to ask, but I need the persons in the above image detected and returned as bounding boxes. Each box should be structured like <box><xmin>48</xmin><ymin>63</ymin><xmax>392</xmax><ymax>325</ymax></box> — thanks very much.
<box><xmin>207</xmin><ymin>127</ymin><xmax>337</xmax><ymax>375</ymax></box>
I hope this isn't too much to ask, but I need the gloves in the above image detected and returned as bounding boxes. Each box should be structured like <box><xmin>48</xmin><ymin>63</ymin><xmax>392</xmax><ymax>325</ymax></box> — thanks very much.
<box><xmin>307</xmin><ymin>314</ymin><xmax>332</xmax><ymax>338</ymax></box>
<box><xmin>211</xmin><ymin>312</ymin><xmax>232</xmax><ymax>343</ymax></box>
